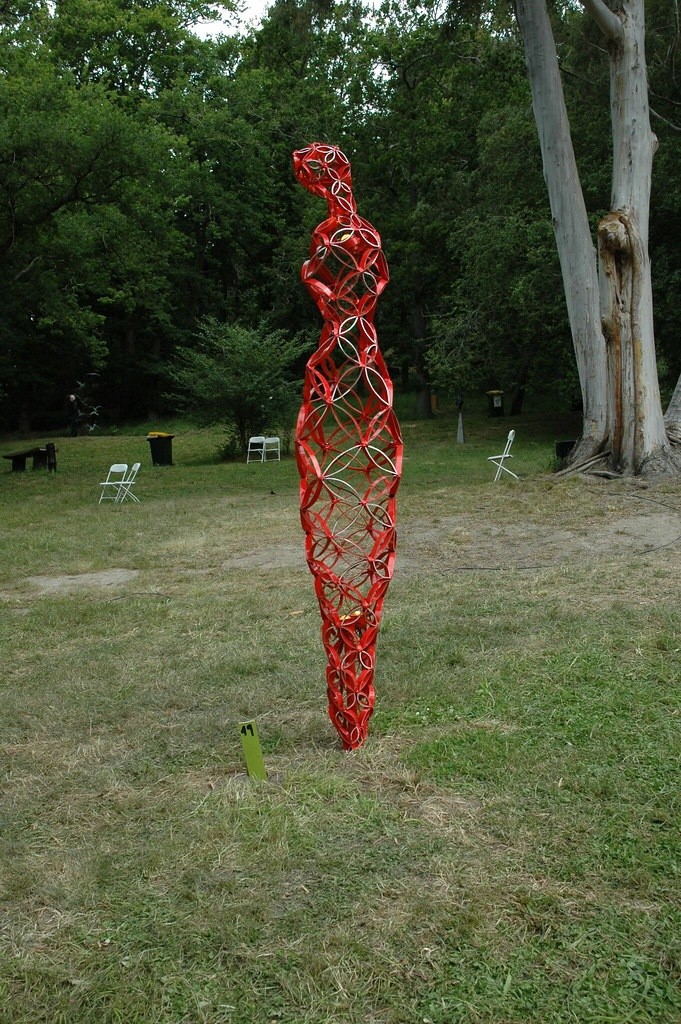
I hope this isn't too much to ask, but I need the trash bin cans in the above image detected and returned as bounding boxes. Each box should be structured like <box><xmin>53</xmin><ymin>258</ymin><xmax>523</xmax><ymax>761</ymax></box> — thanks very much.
<box><xmin>487</xmin><ymin>390</ymin><xmax>505</xmax><ymax>418</ymax></box>
<box><xmin>146</xmin><ymin>435</ymin><xmax>175</xmax><ymax>466</ymax></box>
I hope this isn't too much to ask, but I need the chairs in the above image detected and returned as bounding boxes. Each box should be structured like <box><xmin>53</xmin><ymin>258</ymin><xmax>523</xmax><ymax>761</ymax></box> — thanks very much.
<box><xmin>264</xmin><ymin>437</ymin><xmax>280</xmax><ymax>462</ymax></box>
<box><xmin>115</xmin><ymin>463</ymin><xmax>142</xmax><ymax>503</ymax></box>
<box><xmin>99</xmin><ymin>464</ymin><xmax>129</xmax><ymax>504</ymax></box>
<box><xmin>246</xmin><ymin>436</ymin><xmax>268</xmax><ymax>463</ymax></box>
<box><xmin>487</xmin><ymin>430</ymin><xmax>519</xmax><ymax>482</ymax></box>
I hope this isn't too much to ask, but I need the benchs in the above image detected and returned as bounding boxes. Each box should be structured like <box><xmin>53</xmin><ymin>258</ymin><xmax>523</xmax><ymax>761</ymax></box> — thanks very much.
<box><xmin>3</xmin><ymin>447</ymin><xmax>59</xmax><ymax>472</ymax></box>
